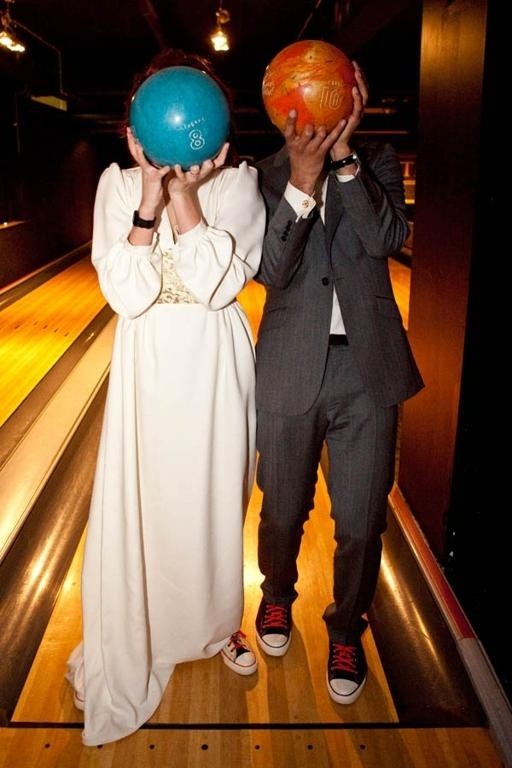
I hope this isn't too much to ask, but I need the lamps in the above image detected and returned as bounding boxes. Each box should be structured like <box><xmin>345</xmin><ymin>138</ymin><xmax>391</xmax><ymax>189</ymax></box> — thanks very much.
<box><xmin>28</xmin><ymin>47</ymin><xmax>70</xmax><ymax>112</ymax></box>
<box><xmin>208</xmin><ymin>2</ymin><xmax>237</xmax><ymax>56</ymax></box>
<box><xmin>0</xmin><ymin>12</ymin><xmax>29</xmax><ymax>53</ymax></box>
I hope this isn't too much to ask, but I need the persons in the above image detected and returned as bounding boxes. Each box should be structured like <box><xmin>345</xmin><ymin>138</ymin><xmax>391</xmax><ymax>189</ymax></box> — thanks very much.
<box><xmin>65</xmin><ymin>47</ymin><xmax>261</xmax><ymax>712</ymax></box>
<box><xmin>251</xmin><ymin>59</ymin><xmax>426</xmax><ymax>706</ymax></box>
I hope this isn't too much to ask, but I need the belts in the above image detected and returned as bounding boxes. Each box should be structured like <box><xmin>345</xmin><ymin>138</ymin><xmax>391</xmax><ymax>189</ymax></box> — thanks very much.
<box><xmin>329</xmin><ymin>333</ymin><xmax>348</xmax><ymax>345</ymax></box>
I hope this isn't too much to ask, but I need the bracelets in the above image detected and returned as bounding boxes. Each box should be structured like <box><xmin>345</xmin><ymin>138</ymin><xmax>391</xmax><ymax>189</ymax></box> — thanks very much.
<box><xmin>330</xmin><ymin>147</ymin><xmax>362</xmax><ymax>171</ymax></box>
<box><xmin>132</xmin><ymin>209</ymin><xmax>157</xmax><ymax>231</ymax></box>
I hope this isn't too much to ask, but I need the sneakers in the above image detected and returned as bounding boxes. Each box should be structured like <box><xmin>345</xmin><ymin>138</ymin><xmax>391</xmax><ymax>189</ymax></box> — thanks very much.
<box><xmin>221</xmin><ymin>630</ymin><xmax>258</xmax><ymax>676</ymax></box>
<box><xmin>326</xmin><ymin>640</ymin><xmax>368</xmax><ymax>704</ymax></box>
<box><xmin>255</xmin><ymin>598</ymin><xmax>292</xmax><ymax>656</ymax></box>
